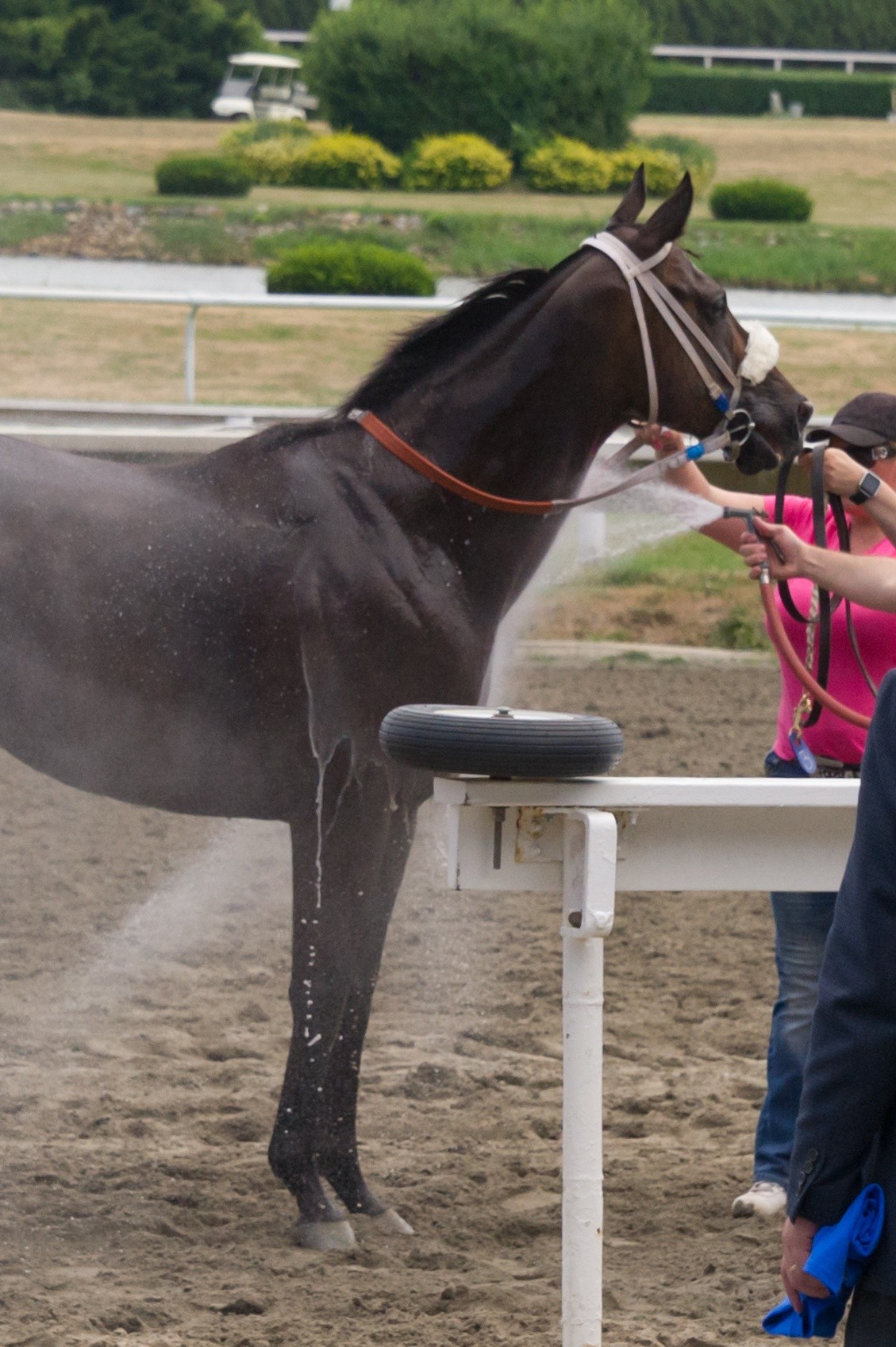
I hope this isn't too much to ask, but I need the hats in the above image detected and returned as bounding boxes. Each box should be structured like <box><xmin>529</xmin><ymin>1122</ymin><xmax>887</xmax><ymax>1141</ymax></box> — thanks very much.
<box><xmin>802</xmin><ymin>390</ymin><xmax>896</xmax><ymax>448</ymax></box>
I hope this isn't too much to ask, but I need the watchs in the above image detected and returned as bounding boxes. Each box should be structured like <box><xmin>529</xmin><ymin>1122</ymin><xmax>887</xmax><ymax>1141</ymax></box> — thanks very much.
<box><xmin>848</xmin><ymin>472</ymin><xmax>881</xmax><ymax>505</ymax></box>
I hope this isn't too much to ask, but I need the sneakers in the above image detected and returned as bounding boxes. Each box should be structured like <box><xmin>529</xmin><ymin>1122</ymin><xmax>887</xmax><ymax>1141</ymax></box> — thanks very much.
<box><xmin>732</xmin><ymin>1179</ymin><xmax>787</xmax><ymax>1217</ymax></box>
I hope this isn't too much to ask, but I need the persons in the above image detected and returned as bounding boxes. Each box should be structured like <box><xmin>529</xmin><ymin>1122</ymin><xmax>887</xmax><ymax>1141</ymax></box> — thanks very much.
<box><xmin>782</xmin><ymin>670</ymin><xmax>896</xmax><ymax>1347</ymax></box>
<box><xmin>637</xmin><ymin>393</ymin><xmax>896</xmax><ymax>1217</ymax></box>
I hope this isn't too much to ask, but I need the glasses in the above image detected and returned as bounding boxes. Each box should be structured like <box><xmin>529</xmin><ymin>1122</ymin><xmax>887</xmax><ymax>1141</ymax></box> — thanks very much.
<box><xmin>847</xmin><ymin>445</ymin><xmax>895</xmax><ymax>470</ymax></box>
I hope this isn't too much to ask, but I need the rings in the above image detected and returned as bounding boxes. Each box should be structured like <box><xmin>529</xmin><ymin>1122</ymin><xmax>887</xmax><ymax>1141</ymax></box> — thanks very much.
<box><xmin>790</xmin><ymin>1264</ymin><xmax>797</xmax><ymax>1271</ymax></box>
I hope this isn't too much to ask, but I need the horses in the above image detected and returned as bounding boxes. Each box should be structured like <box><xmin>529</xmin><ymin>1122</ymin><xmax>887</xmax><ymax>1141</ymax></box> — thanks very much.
<box><xmin>0</xmin><ymin>162</ymin><xmax>816</xmax><ymax>1253</ymax></box>
